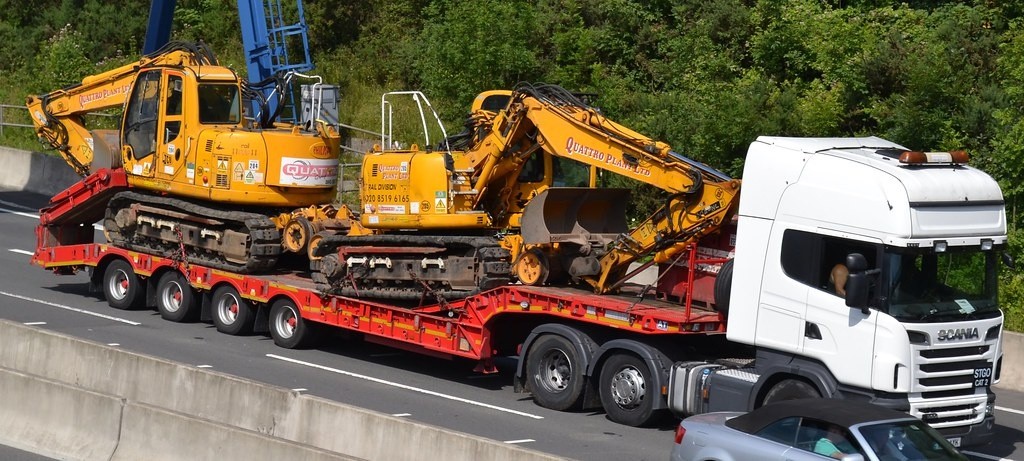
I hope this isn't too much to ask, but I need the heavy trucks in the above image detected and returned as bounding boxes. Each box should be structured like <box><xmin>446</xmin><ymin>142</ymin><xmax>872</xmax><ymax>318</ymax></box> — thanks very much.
<box><xmin>28</xmin><ymin>135</ymin><xmax>1015</xmax><ymax>451</ymax></box>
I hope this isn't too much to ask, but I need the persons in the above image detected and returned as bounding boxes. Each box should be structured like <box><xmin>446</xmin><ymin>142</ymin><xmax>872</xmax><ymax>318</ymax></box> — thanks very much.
<box><xmin>827</xmin><ymin>250</ymin><xmax>871</xmax><ymax>296</ymax></box>
<box><xmin>813</xmin><ymin>424</ymin><xmax>912</xmax><ymax>461</ymax></box>
<box><xmin>166</xmin><ymin>88</ymin><xmax>227</xmax><ymax>133</ymax></box>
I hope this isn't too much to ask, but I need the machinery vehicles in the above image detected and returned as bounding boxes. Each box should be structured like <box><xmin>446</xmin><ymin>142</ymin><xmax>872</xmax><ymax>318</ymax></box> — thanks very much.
<box><xmin>305</xmin><ymin>82</ymin><xmax>742</xmax><ymax>304</ymax></box>
<box><xmin>25</xmin><ymin>0</ymin><xmax>355</xmax><ymax>278</ymax></box>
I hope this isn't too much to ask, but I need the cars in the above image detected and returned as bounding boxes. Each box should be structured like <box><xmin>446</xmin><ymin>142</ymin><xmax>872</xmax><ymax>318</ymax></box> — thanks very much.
<box><xmin>668</xmin><ymin>398</ymin><xmax>969</xmax><ymax>461</ymax></box>
